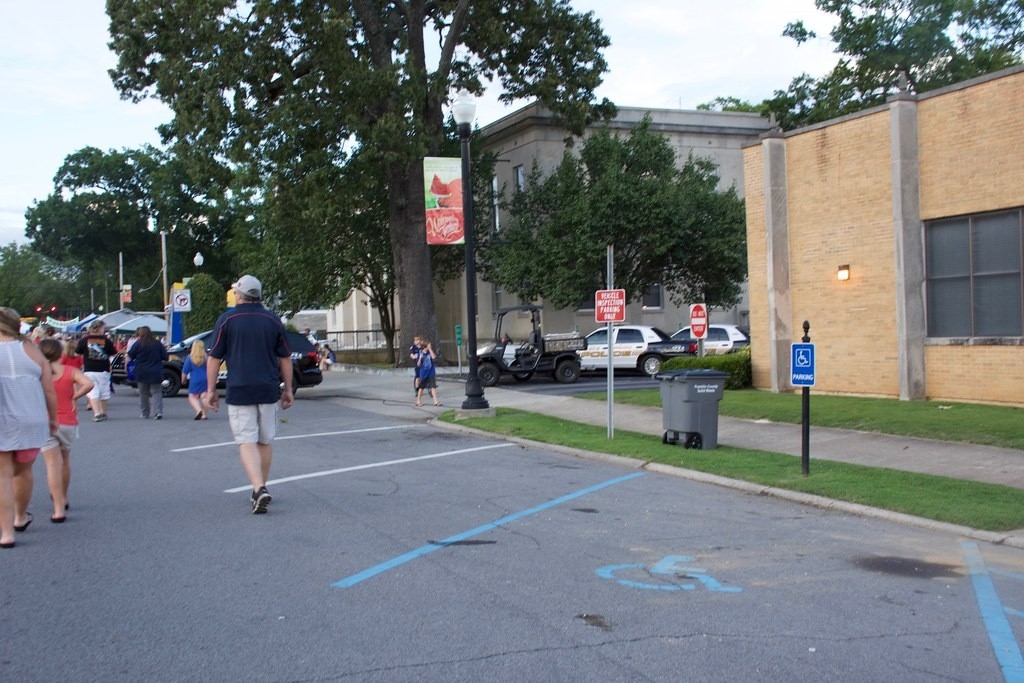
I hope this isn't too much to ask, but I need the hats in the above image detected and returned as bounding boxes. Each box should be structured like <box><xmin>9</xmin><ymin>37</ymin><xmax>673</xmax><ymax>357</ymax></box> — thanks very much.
<box><xmin>231</xmin><ymin>275</ymin><xmax>262</xmax><ymax>298</ymax></box>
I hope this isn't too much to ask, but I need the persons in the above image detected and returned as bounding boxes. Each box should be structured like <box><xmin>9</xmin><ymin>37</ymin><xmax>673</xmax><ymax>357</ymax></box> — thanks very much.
<box><xmin>0</xmin><ymin>306</ymin><xmax>59</xmax><ymax>548</ymax></box>
<box><xmin>39</xmin><ymin>339</ymin><xmax>95</xmax><ymax>523</ymax></box>
<box><xmin>74</xmin><ymin>321</ymin><xmax>119</xmax><ymax>422</ymax></box>
<box><xmin>206</xmin><ymin>274</ymin><xmax>294</xmax><ymax>514</ymax></box>
<box><xmin>409</xmin><ymin>336</ymin><xmax>445</xmax><ymax>407</ymax></box>
<box><xmin>304</xmin><ymin>328</ymin><xmax>336</xmax><ymax>371</ymax></box>
<box><xmin>20</xmin><ymin>321</ymin><xmax>169</xmax><ymax>410</ymax></box>
<box><xmin>181</xmin><ymin>339</ymin><xmax>208</xmax><ymax>420</ymax></box>
<box><xmin>127</xmin><ymin>326</ymin><xmax>169</xmax><ymax>420</ymax></box>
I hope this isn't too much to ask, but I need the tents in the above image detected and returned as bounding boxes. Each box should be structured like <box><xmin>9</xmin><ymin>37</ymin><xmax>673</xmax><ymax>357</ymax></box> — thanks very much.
<box><xmin>110</xmin><ymin>314</ymin><xmax>168</xmax><ymax>336</ymax></box>
<box><xmin>67</xmin><ymin>313</ymin><xmax>98</xmax><ymax>331</ymax></box>
<box><xmin>80</xmin><ymin>308</ymin><xmax>141</xmax><ymax>329</ymax></box>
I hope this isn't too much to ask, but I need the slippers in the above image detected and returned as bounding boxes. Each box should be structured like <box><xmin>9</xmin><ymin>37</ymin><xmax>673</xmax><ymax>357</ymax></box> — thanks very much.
<box><xmin>0</xmin><ymin>542</ymin><xmax>15</xmax><ymax>548</ymax></box>
<box><xmin>50</xmin><ymin>493</ymin><xmax>69</xmax><ymax>510</ymax></box>
<box><xmin>15</xmin><ymin>511</ymin><xmax>33</xmax><ymax>532</ymax></box>
<box><xmin>416</xmin><ymin>402</ymin><xmax>423</xmax><ymax>406</ymax></box>
<box><xmin>50</xmin><ymin>515</ymin><xmax>66</xmax><ymax>524</ymax></box>
<box><xmin>434</xmin><ymin>402</ymin><xmax>442</xmax><ymax>406</ymax></box>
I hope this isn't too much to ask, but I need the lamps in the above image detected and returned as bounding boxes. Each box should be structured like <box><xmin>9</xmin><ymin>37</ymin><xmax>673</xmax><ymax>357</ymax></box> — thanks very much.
<box><xmin>838</xmin><ymin>264</ymin><xmax>849</xmax><ymax>280</ymax></box>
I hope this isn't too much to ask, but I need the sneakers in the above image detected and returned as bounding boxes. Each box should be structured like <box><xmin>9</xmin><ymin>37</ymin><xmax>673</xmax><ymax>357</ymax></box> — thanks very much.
<box><xmin>249</xmin><ymin>484</ymin><xmax>271</xmax><ymax>513</ymax></box>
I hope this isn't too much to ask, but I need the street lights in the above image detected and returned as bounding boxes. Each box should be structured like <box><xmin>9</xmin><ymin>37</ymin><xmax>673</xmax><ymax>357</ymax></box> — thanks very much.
<box><xmin>161</xmin><ymin>231</ymin><xmax>204</xmax><ymax>322</ymax></box>
<box><xmin>90</xmin><ymin>287</ymin><xmax>104</xmax><ymax>314</ymax></box>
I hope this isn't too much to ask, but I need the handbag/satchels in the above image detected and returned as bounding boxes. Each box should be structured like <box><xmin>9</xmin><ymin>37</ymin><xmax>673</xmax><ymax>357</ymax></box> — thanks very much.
<box><xmin>127</xmin><ymin>359</ymin><xmax>137</xmax><ymax>381</ymax></box>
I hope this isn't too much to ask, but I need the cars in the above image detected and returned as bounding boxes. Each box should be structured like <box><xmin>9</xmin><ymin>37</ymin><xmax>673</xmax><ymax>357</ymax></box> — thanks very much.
<box><xmin>670</xmin><ymin>324</ymin><xmax>751</xmax><ymax>358</ymax></box>
<box><xmin>576</xmin><ymin>324</ymin><xmax>698</xmax><ymax>377</ymax></box>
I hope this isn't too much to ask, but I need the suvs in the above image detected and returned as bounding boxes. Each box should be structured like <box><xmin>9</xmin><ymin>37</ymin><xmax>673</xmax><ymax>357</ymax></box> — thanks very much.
<box><xmin>160</xmin><ymin>329</ymin><xmax>323</xmax><ymax>398</ymax></box>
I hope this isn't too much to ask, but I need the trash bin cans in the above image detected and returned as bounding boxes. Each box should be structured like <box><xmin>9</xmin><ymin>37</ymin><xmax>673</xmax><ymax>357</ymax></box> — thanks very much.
<box><xmin>656</xmin><ymin>369</ymin><xmax>731</xmax><ymax>450</ymax></box>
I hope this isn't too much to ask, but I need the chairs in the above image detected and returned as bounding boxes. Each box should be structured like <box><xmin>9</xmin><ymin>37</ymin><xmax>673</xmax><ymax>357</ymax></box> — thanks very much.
<box><xmin>515</xmin><ymin>332</ymin><xmax>535</xmax><ymax>354</ymax></box>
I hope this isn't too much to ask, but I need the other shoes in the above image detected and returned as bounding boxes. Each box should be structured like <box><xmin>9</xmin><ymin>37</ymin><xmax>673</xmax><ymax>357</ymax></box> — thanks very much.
<box><xmin>200</xmin><ymin>416</ymin><xmax>207</xmax><ymax>419</ymax></box>
<box><xmin>154</xmin><ymin>413</ymin><xmax>162</xmax><ymax>420</ymax></box>
<box><xmin>101</xmin><ymin>414</ymin><xmax>107</xmax><ymax>420</ymax></box>
<box><xmin>86</xmin><ymin>404</ymin><xmax>92</xmax><ymax>410</ymax></box>
<box><xmin>140</xmin><ymin>412</ymin><xmax>148</xmax><ymax>418</ymax></box>
<box><xmin>194</xmin><ymin>410</ymin><xmax>202</xmax><ymax>420</ymax></box>
<box><xmin>94</xmin><ymin>414</ymin><xmax>101</xmax><ymax>421</ymax></box>
